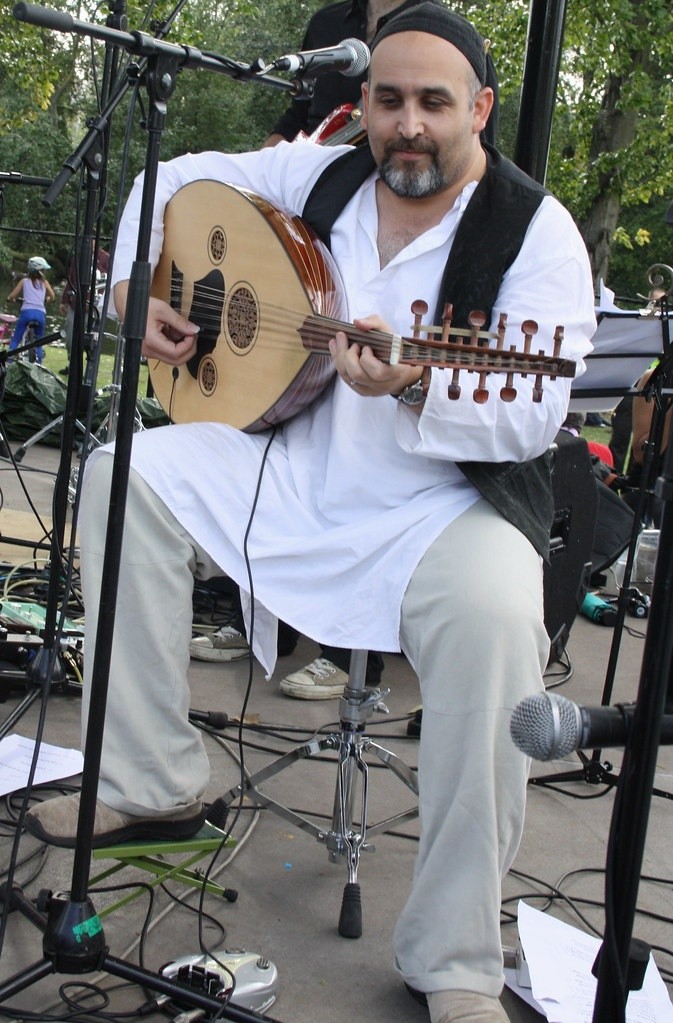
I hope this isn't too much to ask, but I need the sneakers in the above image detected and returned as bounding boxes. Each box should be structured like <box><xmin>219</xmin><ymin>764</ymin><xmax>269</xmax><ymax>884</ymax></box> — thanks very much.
<box><xmin>22</xmin><ymin>787</ymin><xmax>204</xmax><ymax>848</ymax></box>
<box><xmin>279</xmin><ymin>655</ymin><xmax>380</xmax><ymax>701</ymax></box>
<box><xmin>403</xmin><ymin>981</ymin><xmax>511</xmax><ymax>1023</ymax></box>
<box><xmin>189</xmin><ymin>625</ymin><xmax>255</xmax><ymax>661</ymax></box>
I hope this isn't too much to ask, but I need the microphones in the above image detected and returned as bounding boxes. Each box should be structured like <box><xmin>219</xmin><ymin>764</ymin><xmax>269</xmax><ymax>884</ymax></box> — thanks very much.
<box><xmin>271</xmin><ymin>38</ymin><xmax>370</xmax><ymax>77</ymax></box>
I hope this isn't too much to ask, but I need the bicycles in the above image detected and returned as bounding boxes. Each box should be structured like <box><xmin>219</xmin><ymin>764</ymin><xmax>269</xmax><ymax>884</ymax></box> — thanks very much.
<box><xmin>8</xmin><ymin>297</ymin><xmax>45</xmax><ymax>362</ymax></box>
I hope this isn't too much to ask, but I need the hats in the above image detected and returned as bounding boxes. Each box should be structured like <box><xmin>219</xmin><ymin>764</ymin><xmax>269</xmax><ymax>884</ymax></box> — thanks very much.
<box><xmin>370</xmin><ymin>4</ymin><xmax>486</xmax><ymax>88</ymax></box>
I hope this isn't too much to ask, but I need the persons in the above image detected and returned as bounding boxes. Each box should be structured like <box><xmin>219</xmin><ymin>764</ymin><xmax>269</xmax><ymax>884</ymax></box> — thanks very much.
<box><xmin>20</xmin><ymin>0</ymin><xmax>601</xmax><ymax>1023</ymax></box>
<box><xmin>5</xmin><ymin>255</ymin><xmax>55</xmax><ymax>365</ymax></box>
<box><xmin>647</xmin><ymin>287</ymin><xmax>668</xmax><ymax>310</ymax></box>
<box><xmin>189</xmin><ymin>0</ymin><xmax>442</xmax><ymax>702</ymax></box>
<box><xmin>58</xmin><ymin>234</ymin><xmax>111</xmax><ymax>375</ymax></box>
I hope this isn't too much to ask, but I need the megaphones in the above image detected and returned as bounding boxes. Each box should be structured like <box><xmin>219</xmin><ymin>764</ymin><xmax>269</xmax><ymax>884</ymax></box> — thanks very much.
<box><xmin>509</xmin><ymin>692</ymin><xmax>673</xmax><ymax>762</ymax></box>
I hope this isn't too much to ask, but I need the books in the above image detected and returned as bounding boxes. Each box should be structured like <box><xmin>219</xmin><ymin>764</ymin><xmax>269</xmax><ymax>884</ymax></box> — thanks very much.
<box><xmin>567</xmin><ymin>275</ymin><xmax>673</xmax><ymax>413</ymax></box>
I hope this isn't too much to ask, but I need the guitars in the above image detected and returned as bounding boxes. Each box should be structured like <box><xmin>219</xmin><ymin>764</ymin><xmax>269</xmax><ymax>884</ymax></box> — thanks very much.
<box><xmin>147</xmin><ymin>179</ymin><xmax>577</xmax><ymax>433</ymax></box>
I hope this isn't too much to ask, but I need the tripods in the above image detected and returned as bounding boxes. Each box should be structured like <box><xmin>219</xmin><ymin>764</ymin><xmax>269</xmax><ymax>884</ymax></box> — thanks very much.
<box><xmin>0</xmin><ymin>0</ymin><xmax>283</xmax><ymax>1023</ymax></box>
<box><xmin>527</xmin><ymin>295</ymin><xmax>673</xmax><ymax>800</ymax></box>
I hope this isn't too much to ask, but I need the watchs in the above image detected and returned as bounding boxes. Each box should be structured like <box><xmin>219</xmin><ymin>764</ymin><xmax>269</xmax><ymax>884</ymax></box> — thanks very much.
<box><xmin>396</xmin><ymin>377</ymin><xmax>429</xmax><ymax>406</ymax></box>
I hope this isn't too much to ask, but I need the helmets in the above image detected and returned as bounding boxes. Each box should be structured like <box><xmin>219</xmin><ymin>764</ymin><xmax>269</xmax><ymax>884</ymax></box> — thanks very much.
<box><xmin>29</xmin><ymin>257</ymin><xmax>51</xmax><ymax>270</ymax></box>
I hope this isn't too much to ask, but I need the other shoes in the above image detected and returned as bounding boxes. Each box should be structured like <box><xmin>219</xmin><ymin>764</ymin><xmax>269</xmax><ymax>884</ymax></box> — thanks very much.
<box><xmin>59</xmin><ymin>367</ymin><xmax>68</xmax><ymax>376</ymax></box>
